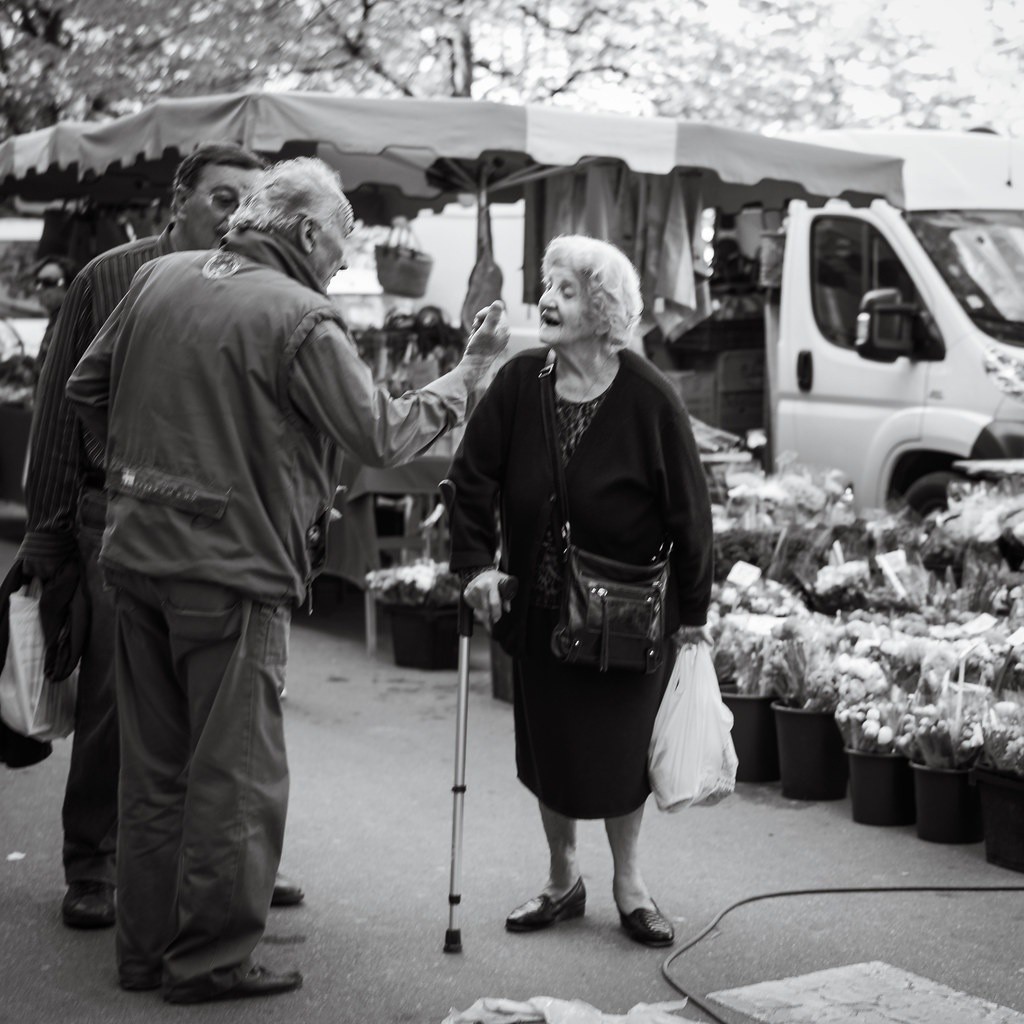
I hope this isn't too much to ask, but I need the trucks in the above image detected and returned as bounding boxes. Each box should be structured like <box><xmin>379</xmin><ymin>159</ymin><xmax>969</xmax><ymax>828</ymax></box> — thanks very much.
<box><xmin>504</xmin><ymin>126</ymin><xmax>1024</xmax><ymax>519</ymax></box>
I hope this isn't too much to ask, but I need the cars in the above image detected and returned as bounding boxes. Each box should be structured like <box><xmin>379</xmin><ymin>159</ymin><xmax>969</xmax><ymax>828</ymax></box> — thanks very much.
<box><xmin>0</xmin><ymin>217</ymin><xmax>49</xmax><ymax>504</ymax></box>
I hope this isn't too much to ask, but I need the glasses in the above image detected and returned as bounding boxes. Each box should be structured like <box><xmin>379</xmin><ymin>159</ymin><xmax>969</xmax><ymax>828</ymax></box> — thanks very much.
<box><xmin>189</xmin><ymin>188</ymin><xmax>237</xmax><ymax>215</ymax></box>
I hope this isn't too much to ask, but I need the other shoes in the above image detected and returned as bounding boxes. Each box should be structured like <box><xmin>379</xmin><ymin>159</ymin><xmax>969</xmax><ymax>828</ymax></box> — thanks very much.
<box><xmin>506</xmin><ymin>875</ymin><xmax>586</xmax><ymax>931</ymax></box>
<box><xmin>170</xmin><ymin>963</ymin><xmax>303</xmax><ymax>1004</ymax></box>
<box><xmin>61</xmin><ymin>879</ymin><xmax>116</xmax><ymax>929</ymax></box>
<box><xmin>271</xmin><ymin>873</ymin><xmax>304</xmax><ymax>905</ymax></box>
<box><xmin>617</xmin><ymin>898</ymin><xmax>674</xmax><ymax>946</ymax></box>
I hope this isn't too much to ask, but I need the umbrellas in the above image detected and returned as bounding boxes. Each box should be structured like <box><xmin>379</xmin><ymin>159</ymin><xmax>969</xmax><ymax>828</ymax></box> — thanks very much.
<box><xmin>0</xmin><ymin>92</ymin><xmax>905</xmax><ymax>299</ymax></box>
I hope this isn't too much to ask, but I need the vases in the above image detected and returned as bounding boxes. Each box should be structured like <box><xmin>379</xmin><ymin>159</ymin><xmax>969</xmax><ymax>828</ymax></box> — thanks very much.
<box><xmin>391</xmin><ymin>603</ymin><xmax>474</xmax><ymax>671</ymax></box>
<box><xmin>715</xmin><ymin>690</ymin><xmax>1024</xmax><ymax>874</ymax></box>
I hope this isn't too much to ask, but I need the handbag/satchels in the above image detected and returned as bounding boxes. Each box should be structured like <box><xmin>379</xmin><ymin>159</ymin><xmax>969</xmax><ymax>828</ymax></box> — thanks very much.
<box><xmin>550</xmin><ymin>545</ymin><xmax>671</xmax><ymax>673</ymax></box>
<box><xmin>647</xmin><ymin>641</ymin><xmax>739</xmax><ymax>813</ymax></box>
<box><xmin>0</xmin><ymin>586</ymin><xmax>81</xmax><ymax>744</ymax></box>
<box><xmin>377</xmin><ymin>223</ymin><xmax>432</xmax><ymax>296</ymax></box>
<box><xmin>0</xmin><ymin>553</ymin><xmax>50</xmax><ymax>772</ymax></box>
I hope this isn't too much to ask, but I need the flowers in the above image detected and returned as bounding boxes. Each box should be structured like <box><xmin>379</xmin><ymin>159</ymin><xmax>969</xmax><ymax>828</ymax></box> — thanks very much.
<box><xmin>694</xmin><ymin>470</ymin><xmax>1024</xmax><ymax>773</ymax></box>
<box><xmin>361</xmin><ymin>558</ymin><xmax>465</xmax><ymax>609</ymax></box>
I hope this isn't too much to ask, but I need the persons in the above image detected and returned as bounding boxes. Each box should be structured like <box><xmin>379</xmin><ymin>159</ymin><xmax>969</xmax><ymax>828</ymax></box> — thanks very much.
<box><xmin>0</xmin><ymin>144</ymin><xmax>304</xmax><ymax>931</ymax></box>
<box><xmin>66</xmin><ymin>156</ymin><xmax>512</xmax><ymax>1006</ymax></box>
<box><xmin>441</xmin><ymin>233</ymin><xmax>714</xmax><ymax>947</ymax></box>
